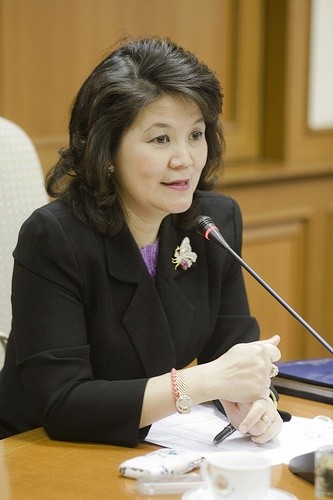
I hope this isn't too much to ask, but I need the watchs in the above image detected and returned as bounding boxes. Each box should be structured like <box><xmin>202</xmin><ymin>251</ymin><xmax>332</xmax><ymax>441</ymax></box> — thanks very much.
<box><xmin>177</xmin><ymin>370</ymin><xmax>192</xmax><ymax>414</ymax></box>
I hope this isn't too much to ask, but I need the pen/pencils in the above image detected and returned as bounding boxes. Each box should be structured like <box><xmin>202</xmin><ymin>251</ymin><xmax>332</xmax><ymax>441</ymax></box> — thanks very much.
<box><xmin>211</xmin><ymin>422</ymin><xmax>236</xmax><ymax>444</ymax></box>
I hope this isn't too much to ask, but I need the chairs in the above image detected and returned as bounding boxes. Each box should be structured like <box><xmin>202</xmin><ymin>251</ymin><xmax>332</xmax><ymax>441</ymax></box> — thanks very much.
<box><xmin>0</xmin><ymin>117</ymin><xmax>49</xmax><ymax>369</ymax></box>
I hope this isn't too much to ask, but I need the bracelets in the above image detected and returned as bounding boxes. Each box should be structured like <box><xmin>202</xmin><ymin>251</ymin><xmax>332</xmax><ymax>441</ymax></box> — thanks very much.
<box><xmin>172</xmin><ymin>368</ymin><xmax>182</xmax><ymax>414</ymax></box>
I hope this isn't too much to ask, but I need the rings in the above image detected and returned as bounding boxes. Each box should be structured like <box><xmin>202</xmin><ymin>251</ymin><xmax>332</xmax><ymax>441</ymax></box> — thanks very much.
<box><xmin>262</xmin><ymin>415</ymin><xmax>272</xmax><ymax>426</ymax></box>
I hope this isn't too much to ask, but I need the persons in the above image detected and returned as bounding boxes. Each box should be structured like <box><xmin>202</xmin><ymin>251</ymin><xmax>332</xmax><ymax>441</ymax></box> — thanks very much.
<box><xmin>0</xmin><ymin>37</ymin><xmax>283</xmax><ymax>449</ymax></box>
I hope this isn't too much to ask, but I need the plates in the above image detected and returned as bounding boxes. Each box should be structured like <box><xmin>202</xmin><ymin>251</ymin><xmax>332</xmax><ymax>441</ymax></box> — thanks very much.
<box><xmin>182</xmin><ymin>486</ymin><xmax>297</xmax><ymax>499</ymax></box>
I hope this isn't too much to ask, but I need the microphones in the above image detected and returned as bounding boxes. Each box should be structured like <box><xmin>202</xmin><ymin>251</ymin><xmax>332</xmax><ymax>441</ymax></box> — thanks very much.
<box><xmin>193</xmin><ymin>214</ymin><xmax>333</xmax><ymax>355</ymax></box>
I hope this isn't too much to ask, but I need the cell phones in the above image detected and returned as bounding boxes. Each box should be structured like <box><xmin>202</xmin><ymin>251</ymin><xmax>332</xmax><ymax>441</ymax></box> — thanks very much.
<box><xmin>119</xmin><ymin>448</ymin><xmax>205</xmax><ymax>480</ymax></box>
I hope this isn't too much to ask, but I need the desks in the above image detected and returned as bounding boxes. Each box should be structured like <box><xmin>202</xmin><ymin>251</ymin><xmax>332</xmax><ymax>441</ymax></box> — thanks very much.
<box><xmin>0</xmin><ymin>384</ymin><xmax>333</xmax><ymax>500</ymax></box>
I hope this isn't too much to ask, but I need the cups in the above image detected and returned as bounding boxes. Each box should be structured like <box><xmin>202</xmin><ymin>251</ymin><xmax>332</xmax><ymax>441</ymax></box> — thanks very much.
<box><xmin>313</xmin><ymin>414</ymin><xmax>333</xmax><ymax>500</ymax></box>
<box><xmin>200</xmin><ymin>451</ymin><xmax>276</xmax><ymax>499</ymax></box>
